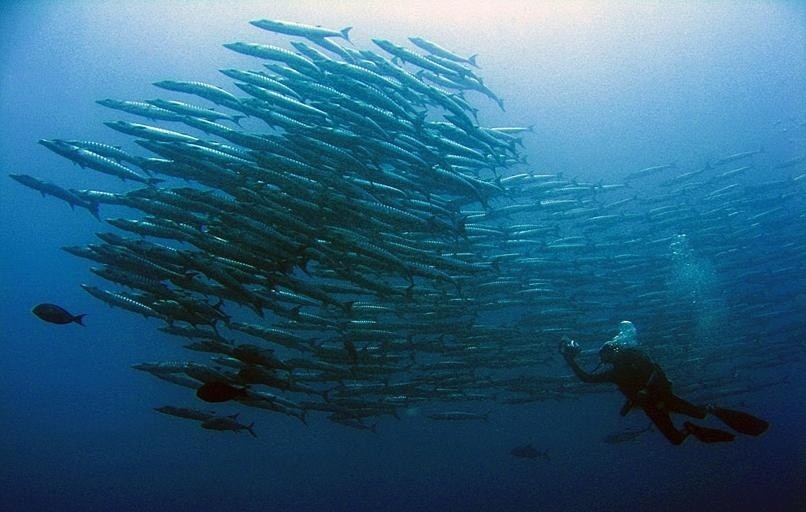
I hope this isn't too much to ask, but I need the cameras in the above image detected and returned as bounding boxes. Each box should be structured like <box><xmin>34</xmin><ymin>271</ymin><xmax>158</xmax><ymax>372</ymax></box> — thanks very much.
<box><xmin>566</xmin><ymin>339</ymin><xmax>582</xmax><ymax>354</ymax></box>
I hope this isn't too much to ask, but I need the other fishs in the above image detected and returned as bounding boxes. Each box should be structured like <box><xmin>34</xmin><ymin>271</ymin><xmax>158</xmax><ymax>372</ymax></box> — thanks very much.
<box><xmin>7</xmin><ymin>17</ymin><xmax>806</xmax><ymax>440</ymax></box>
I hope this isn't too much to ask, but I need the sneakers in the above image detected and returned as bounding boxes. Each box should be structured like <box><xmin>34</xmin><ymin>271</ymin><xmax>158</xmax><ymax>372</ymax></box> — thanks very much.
<box><xmin>684</xmin><ymin>421</ymin><xmax>698</xmax><ymax>435</ymax></box>
<box><xmin>705</xmin><ymin>403</ymin><xmax>716</xmax><ymax>416</ymax></box>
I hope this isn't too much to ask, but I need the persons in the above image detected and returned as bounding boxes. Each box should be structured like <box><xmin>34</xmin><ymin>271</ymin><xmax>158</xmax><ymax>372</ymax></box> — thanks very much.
<box><xmin>563</xmin><ymin>339</ymin><xmax>717</xmax><ymax>445</ymax></box>
